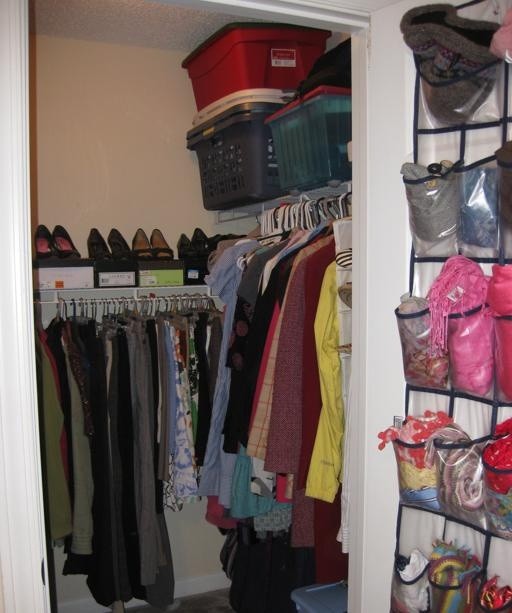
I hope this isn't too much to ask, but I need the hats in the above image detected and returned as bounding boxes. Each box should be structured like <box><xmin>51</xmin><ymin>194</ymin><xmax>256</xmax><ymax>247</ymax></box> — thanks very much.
<box><xmin>399</xmin><ymin>1</ymin><xmax>510</xmax><ymax>129</ymax></box>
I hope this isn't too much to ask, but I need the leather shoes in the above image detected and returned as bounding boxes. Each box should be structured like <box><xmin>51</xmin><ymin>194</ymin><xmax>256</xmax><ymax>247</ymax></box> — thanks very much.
<box><xmin>131</xmin><ymin>227</ymin><xmax>154</xmax><ymax>261</ymax></box>
<box><xmin>107</xmin><ymin>227</ymin><xmax>132</xmax><ymax>261</ymax></box>
<box><xmin>87</xmin><ymin>227</ymin><xmax>114</xmax><ymax>264</ymax></box>
<box><xmin>150</xmin><ymin>228</ymin><xmax>174</xmax><ymax>260</ymax></box>
<box><xmin>51</xmin><ymin>224</ymin><xmax>81</xmax><ymax>258</ymax></box>
<box><xmin>34</xmin><ymin>224</ymin><xmax>54</xmax><ymax>259</ymax></box>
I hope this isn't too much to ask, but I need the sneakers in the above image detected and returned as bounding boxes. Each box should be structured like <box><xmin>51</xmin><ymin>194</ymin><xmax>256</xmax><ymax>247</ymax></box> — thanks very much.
<box><xmin>178</xmin><ymin>227</ymin><xmax>211</xmax><ymax>258</ymax></box>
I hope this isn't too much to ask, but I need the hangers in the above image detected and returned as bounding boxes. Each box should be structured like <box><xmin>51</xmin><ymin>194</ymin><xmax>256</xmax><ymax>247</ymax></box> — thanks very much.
<box><xmin>234</xmin><ymin>192</ymin><xmax>349</xmax><ymax>269</ymax></box>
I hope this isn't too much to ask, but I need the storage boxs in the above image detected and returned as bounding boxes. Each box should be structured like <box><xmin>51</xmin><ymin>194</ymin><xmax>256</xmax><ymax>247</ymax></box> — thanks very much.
<box><xmin>32</xmin><ymin>257</ymin><xmax>186</xmax><ymax>292</ymax></box>
<box><xmin>179</xmin><ymin>20</ymin><xmax>355</xmax><ymax>213</ymax></box>
